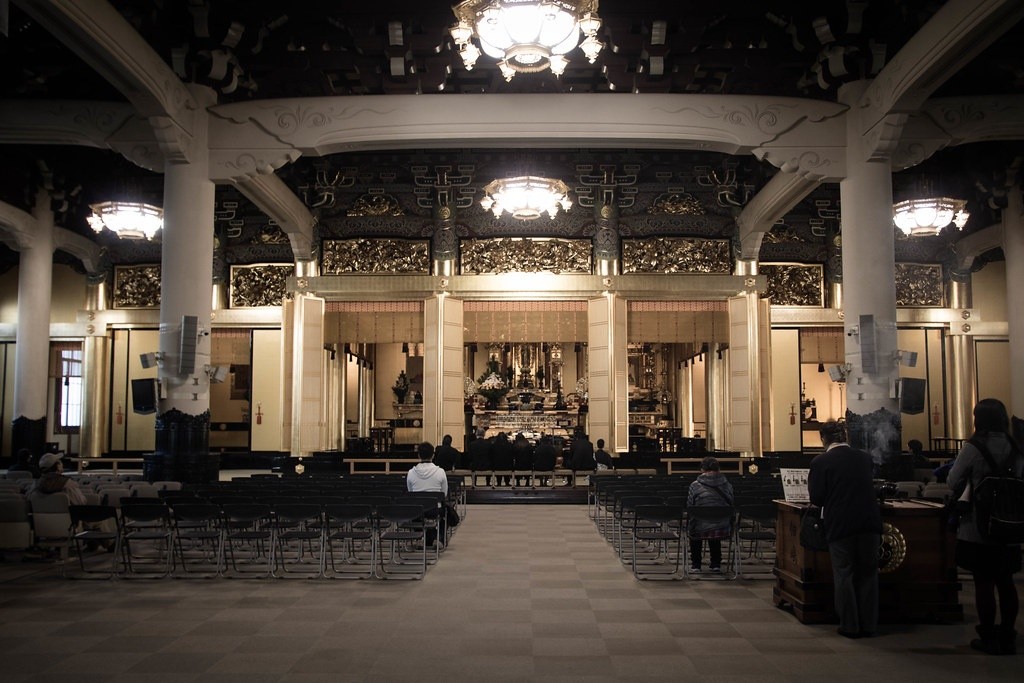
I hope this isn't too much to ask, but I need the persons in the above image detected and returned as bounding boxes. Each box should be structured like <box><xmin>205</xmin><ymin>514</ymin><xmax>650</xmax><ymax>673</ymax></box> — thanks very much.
<box><xmin>595</xmin><ymin>439</ymin><xmax>612</xmax><ymax>469</ymax></box>
<box><xmin>492</xmin><ymin>431</ymin><xmax>514</xmax><ymax>486</ymax></box>
<box><xmin>694</xmin><ymin>434</ymin><xmax>701</xmax><ymax>438</ymax></box>
<box><xmin>535</xmin><ymin>430</ymin><xmax>552</xmax><ymax>447</ymax></box>
<box><xmin>946</xmin><ymin>398</ymin><xmax>1024</xmax><ymax>653</ymax></box>
<box><xmin>893</xmin><ymin>439</ymin><xmax>932</xmax><ymax>485</ymax></box>
<box><xmin>533</xmin><ymin>438</ymin><xmax>557</xmax><ymax>486</ymax></box>
<box><xmin>433</xmin><ymin>434</ymin><xmax>460</xmax><ymax>471</ymax></box>
<box><xmin>407</xmin><ymin>441</ymin><xmax>448</xmax><ymax>549</ymax></box>
<box><xmin>564</xmin><ymin>430</ymin><xmax>595</xmax><ymax>486</ymax></box>
<box><xmin>623</xmin><ymin>439</ymin><xmax>666</xmax><ymax>474</ymax></box>
<box><xmin>686</xmin><ymin>457</ymin><xmax>734</xmax><ymax>571</ymax></box>
<box><xmin>27</xmin><ymin>452</ymin><xmax>116</xmax><ymax>553</ymax></box>
<box><xmin>807</xmin><ymin>421</ymin><xmax>882</xmax><ymax>639</ymax></box>
<box><xmin>514</xmin><ymin>432</ymin><xmax>533</xmax><ymax>487</ymax></box>
<box><xmin>468</xmin><ymin>427</ymin><xmax>493</xmax><ymax>486</ymax></box>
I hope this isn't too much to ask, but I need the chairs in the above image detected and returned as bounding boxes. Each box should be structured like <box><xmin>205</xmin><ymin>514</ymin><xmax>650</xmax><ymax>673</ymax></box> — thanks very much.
<box><xmin>0</xmin><ymin>447</ymin><xmax>950</xmax><ymax>605</ymax></box>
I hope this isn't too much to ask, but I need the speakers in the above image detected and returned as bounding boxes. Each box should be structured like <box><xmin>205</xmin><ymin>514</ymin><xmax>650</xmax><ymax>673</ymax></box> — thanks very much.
<box><xmin>898</xmin><ymin>377</ymin><xmax>927</xmax><ymax>415</ymax></box>
<box><xmin>132</xmin><ymin>378</ymin><xmax>156</xmax><ymax>414</ymax></box>
<box><xmin>827</xmin><ymin>364</ymin><xmax>843</xmax><ymax>382</ymax></box>
<box><xmin>901</xmin><ymin>351</ymin><xmax>917</xmax><ymax>367</ymax></box>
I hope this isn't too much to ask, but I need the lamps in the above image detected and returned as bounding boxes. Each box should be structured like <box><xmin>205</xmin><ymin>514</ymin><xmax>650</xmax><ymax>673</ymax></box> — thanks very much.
<box><xmin>858</xmin><ymin>314</ymin><xmax>878</xmax><ymax>375</ymax></box>
<box><xmin>140</xmin><ymin>352</ymin><xmax>165</xmax><ymax>369</ymax></box>
<box><xmin>209</xmin><ymin>366</ymin><xmax>230</xmax><ymax>384</ymax></box>
<box><xmin>86</xmin><ymin>163</ymin><xmax>164</xmax><ymax>241</ymax></box>
<box><xmin>448</xmin><ymin>0</ymin><xmax>604</xmax><ymax>83</ymax></box>
<box><xmin>828</xmin><ymin>363</ymin><xmax>851</xmax><ymax>383</ymax></box>
<box><xmin>892</xmin><ymin>156</ymin><xmax>972</xmax><ymax>236</ymax></box>
<box><xmin>402</xmin><ymin>343</ymin><xmax>409</xmax><ymax>353</ymax></box>
<box><xmin>893</xmin><ymin>350</ymin><xmax>918</xmax><ymax>367</ymax></box>
<box><xmin>478</xmin><ymin>152</ymin><xmax>573</xmax><ymax>220</ymax></box>
<box><xmin>344</xmin><ymin>343</ymin><xmax>351</xmax><ymax>353</ymax></box>
<box><xmin>818</xmin><ymin>364</ymin><xmax>825</xmax><ymax>372</ymax></box>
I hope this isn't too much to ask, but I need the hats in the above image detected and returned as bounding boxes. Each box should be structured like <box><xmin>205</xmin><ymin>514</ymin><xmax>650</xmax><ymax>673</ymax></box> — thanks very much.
<box><xmin>39</xmin><ymin>452</ymin><xmax>64</xmax><ymax>472</ymax></box>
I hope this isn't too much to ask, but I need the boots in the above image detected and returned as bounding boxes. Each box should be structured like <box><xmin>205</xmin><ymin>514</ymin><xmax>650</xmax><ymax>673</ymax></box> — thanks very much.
<box><xmin>971</xmin><ymin>623</ymin><xmax>1000</xmax><ymax>655</ymax></box>
<box><xmin>1001</xmin><ymin>625</ymin><xmax>1018</xmax><ymax>656</ymax></box>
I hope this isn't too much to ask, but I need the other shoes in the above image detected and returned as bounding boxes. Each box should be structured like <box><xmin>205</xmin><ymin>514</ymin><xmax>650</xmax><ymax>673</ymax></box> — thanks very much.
<box><xmin>486</xmin><ymin>481</ymin><xmax>490</xmax><ymax>486</ymax></box>
<box><xmin>506</xmin><ymin>482</ymin><xmax>512</xmax><ymax>486</ymax></box>
<box><xmin>525</xmin><ymin>482</ymin><xmax>529</xmax><ymax>487</ymax></box>
<box><xmin>433</xmin><ymin>540</ymin><xmax>443</xmax><ymax>550</ymax></box>
<box><xmin>88</xmin><ymin>542</ymin><xmax>100</xmax><ymax>552</ymax></box>
<box><xmin>540</xmin><ymin>482</ymin><xmax>548</xmax><ymax>487</ymax></box>
<box><xmin>564</xmin><ymin>483</ymin><xmax>572</xmax><ymax>487</ymax></box>
<box><xmin>691</xmin><ymin>565</ymin><xmax>700</xmax><ymax>572</ymax></box>
<box><xmin>497</xmin><ymin>482</ymin><xmax>501</xmax><ymax>487</ymax></box>
<box><xmin>516</xmin><ymin>481</ymin><xmax>520</xmax><ymax>487</ymax></box>
<box><xmin>708</xmin><ymin>564</ymin><xmax>720</xmax><ymax>572</ymax></box>
<box><xmin>416</xmin><ymin>541</ymin><xmax>424</xmax><ymax>550</ymax></box>
<box><xmin>108</xmin><ymin>538</ymin><xmax>126</xmax><ymax>553</ymax></box>
<box><xmin>838</xmin><ymin>626</ymin><xmax>862</xmax><ymax>639</ymax></box>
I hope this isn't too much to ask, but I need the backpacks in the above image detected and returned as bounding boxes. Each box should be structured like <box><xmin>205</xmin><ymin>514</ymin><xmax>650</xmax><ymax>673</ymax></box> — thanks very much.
<box><xmin>970</xmin><ymin>441</ymin><xmax>1024</xmax><ymax>545</ymax></box>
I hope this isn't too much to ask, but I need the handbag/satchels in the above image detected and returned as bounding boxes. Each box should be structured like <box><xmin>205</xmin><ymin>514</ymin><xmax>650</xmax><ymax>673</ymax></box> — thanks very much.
<box><xmin>798</xmin><ymin>501</ymin><xmax>828</xmax><ymax>551</ymax></box>
<box><xmin>446</xmin><ymin>504</ymin><xmax>460</xmax><ymax>527</ymax></box>
